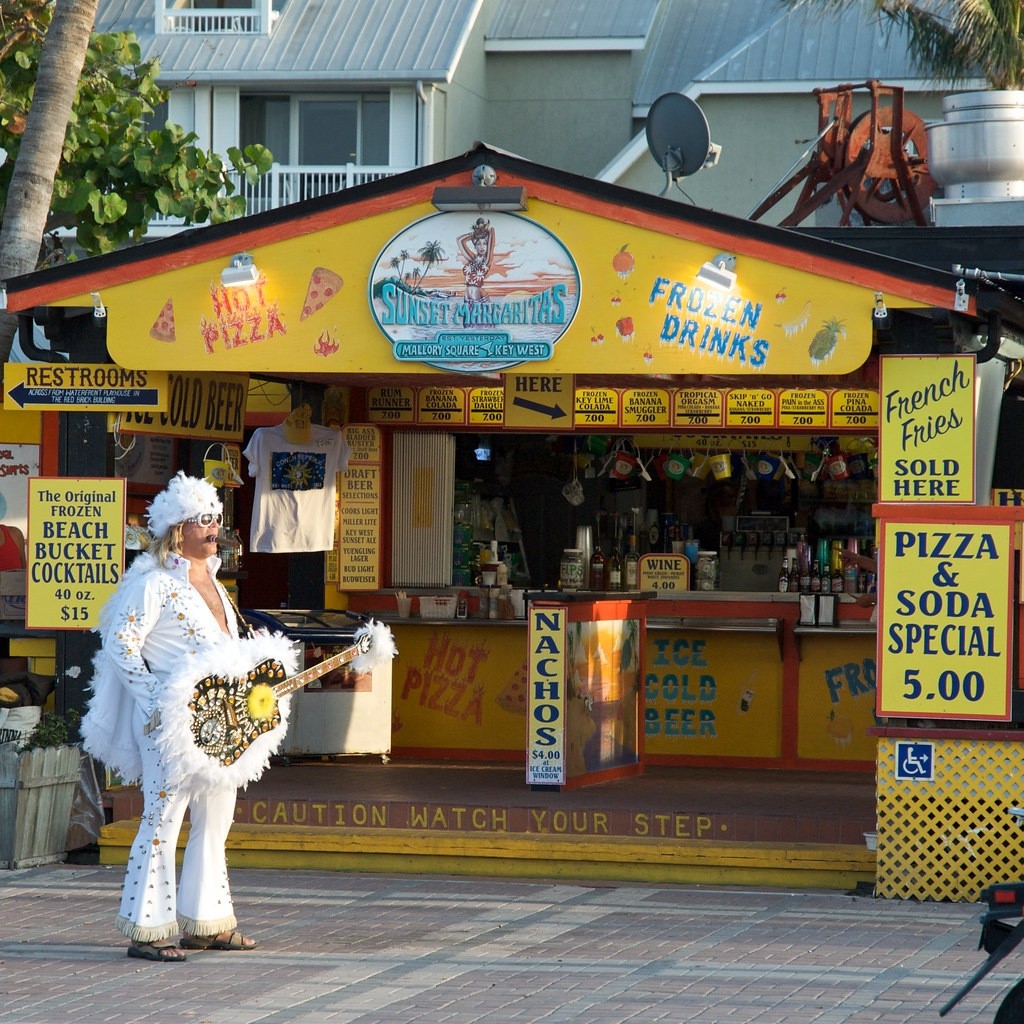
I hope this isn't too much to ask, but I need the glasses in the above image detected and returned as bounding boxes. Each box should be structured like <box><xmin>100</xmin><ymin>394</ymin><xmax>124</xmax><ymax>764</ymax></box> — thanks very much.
<box><xmin>187</xmin><ymin>512</ymin><xmax>224</xmax><ymax>528</ymax></box>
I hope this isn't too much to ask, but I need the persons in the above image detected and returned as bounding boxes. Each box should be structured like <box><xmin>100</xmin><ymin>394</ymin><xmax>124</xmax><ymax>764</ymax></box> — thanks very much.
<box><xmin>695</xmin><ymin>482</ymin><xmax>737</xmax><ymax>551</ymax></box>
<box><xmin>0</xmin><ymin>525</ymin><xmax>26</xmax><ymax>573</ymax></box>
<box><xmin>78</xmin><ymin>472</ymin><xmax>258</xmax><ymax>963</ymax></box>
<box><xmin>831</xmin><ymin>546</ymin><xmax>877</xmax><ymax>626</ymax></box>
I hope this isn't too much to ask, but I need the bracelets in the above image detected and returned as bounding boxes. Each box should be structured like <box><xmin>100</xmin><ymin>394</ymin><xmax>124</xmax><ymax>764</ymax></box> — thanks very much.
<box><xmin>854</xmin><ymin>553</ymin><xmax>860</xmax><ymax>564</ymax></box>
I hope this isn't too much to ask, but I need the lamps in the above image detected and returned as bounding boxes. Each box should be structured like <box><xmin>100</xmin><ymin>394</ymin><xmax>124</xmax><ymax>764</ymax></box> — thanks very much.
<box><xmin>695</xmin><ymin>253</ymin><xmax>736</xmax><ymax>292</ymax></box>
<box><xmin>431</xmin><ymin>163</ymin><xmax>528</xmax><ymax>212</ymax></box>
<box><xmin>221</xmin><ymin>253</ymin><xmax>259</xmax><ymax>288</ymax></box>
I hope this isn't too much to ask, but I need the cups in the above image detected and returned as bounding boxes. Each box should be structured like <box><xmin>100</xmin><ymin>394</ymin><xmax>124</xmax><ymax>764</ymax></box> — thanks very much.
<box><xmin>397</xmin><ymin>597</ymin><xmax>411</xmax><ymax>618</ymax></box>
<box><xmin>817</xmin><ymin>538</ymin><xmax>861</xmax><ymax>572</ymax></box>
<box><xmin>562</xmin><ymin>480</ymin><xmax>586</xmax><ymax>506</ymax></box>
<box><xmin>575</xmin><ymin>525</ymin><xmax>594</xmax><ymax>592</ymax></box>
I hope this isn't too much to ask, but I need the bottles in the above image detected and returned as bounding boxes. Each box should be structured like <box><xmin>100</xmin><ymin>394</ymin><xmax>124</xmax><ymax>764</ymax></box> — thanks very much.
<box><xmin>218</xmin><ymin>526</ymin><xmax>243</xmax><ymax>573</ymax></box>
<box><xmin>797</xmin><ymin>534</ymin><xmax>812</xmax><ymax>572</ymax></box>
<box><xmin>783</xmin><ymin>532</ymin><xmax>800</xmax><ymax>575</ymax></box>
<box><xmin>496</xmin><ymin>564</ymin><xmax>507</xmax><ymax>586</ymax></box>
<box><xmin>456</xmin><ymin>588</ymin><xmax>515</xmax><ymax>620</ymax></box>
<box><xmin>592</xmin><ymin>508</ymin><xmax>721</xmax><ymax>591</ymax></box>
<box><xmin>777</xmin><ymin>558</ymin><xmax>878</xmax><ymax>593</ymax></box>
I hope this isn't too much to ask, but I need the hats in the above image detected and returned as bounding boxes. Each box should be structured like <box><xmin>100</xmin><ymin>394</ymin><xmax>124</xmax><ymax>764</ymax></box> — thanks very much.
<box><xmin>147</xmin><ymin>471</ymin><xmax>223</xmax><ymax>536</ymax></box>
<box><xmin>284</xmin><ymin>403</ymin><xmax>314</xmax><ymax>445</ymax></box>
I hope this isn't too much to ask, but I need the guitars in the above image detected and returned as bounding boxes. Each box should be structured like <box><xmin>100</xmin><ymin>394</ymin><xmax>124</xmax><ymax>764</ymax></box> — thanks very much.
<box><xmin>187</xmin><ymin>633</ymin><xmax>374</xmax><ymax>766</ymax></box>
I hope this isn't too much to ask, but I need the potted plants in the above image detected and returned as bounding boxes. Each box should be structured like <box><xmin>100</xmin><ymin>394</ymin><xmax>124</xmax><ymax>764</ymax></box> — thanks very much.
<box><xmin>0</xmin><ymin>709</ymin><xmax>81</xmax><ymax>871</ymax></box>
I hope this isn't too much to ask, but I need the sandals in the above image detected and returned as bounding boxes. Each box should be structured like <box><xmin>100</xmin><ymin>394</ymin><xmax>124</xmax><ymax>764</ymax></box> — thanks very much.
<box><xmin>128</xmin><ymin>937</ymin><xmax>187</xmax><ymax>961</ymax></box>
<box><xmin>180</xmin><ymin>928</ymin><xmax>257</xmax><ymax>951</ymax></box>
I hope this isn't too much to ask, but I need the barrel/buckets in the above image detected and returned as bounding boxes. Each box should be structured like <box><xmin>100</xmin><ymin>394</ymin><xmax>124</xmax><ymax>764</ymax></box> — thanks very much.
<box><xmin>567</xmin><ymin>436</ymin><xmax>879</xmax><ymax>481</ymax></box>
<box><xmin>202</xmin><ymin>442</ymin><xmax>230</xmax><ymax>488</ymax></box>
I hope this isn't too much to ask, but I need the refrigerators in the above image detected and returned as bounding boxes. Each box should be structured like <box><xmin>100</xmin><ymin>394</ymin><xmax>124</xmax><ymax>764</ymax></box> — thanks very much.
<box><xmin>237</xmin><ymin>608</ymin><xmax>392</xmax><ymax>755</ymax></box>
